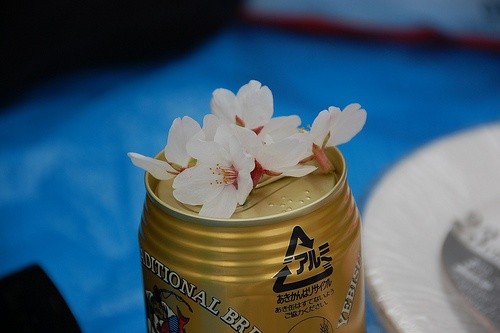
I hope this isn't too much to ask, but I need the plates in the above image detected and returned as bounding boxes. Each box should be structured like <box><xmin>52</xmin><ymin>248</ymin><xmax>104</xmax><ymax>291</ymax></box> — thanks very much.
<box><xmin>362</xmin><ymin>121</ymin><xmax>500</xmax><ymax>333</ymax></box>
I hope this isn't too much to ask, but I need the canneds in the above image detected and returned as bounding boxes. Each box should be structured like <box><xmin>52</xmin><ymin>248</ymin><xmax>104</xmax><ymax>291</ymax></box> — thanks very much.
<box><xmin>139</xmin><ymin>128</ymin><xmax>365</xmax><ymax>333</ymax></box>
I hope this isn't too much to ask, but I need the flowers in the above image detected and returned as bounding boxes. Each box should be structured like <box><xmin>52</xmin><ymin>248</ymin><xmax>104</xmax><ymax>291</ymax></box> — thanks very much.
<box><xmin>126</xmin><ymin>80</ymin><xmax>367</xmax><ymax>219</ymax></box>
